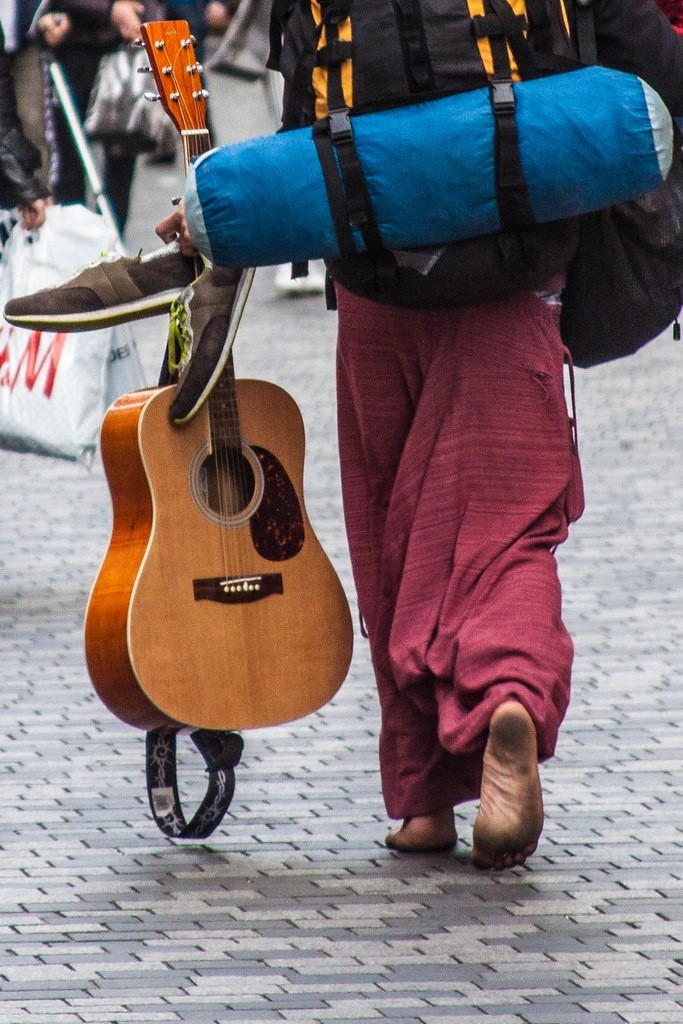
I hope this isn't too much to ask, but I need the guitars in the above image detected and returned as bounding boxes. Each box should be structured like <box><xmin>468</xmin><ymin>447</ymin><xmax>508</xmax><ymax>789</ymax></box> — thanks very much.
<box><xmin>79</xmin><ymin>23</ymin><xmax>357</xmax><ymax>728</ymax></box>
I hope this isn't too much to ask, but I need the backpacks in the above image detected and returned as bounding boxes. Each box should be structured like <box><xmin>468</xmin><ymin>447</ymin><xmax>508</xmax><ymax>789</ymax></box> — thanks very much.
<box><xmin>560</xmin><ymin>1</ymin><xmax>683</xmax><ymax>368</ymax></box>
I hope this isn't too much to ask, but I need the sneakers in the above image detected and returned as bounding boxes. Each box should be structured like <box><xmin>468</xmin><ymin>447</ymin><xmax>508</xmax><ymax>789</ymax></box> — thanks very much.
<box><xmin>165</xmin><ymin>262</ymin><xmax>258</xmax><ymax>425</ymax></box>
<box><xmin>3</xmin><ymin>239</ymin><xmax>206</xmax><ymax>334</ymax></box>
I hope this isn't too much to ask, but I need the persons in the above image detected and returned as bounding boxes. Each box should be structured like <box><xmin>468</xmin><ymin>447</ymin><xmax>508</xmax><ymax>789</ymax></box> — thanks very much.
<box><xmin>0</xmin><ymin>0</ymin><xmax>327</xmax><ymax>293</ymax></box>
<box><xmin>156</xmin><ymin>0</ymin><xmax>683</xmax><ymax>873</ymax></box>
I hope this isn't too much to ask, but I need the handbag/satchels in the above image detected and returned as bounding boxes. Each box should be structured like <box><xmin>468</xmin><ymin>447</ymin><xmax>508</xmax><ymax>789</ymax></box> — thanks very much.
<box><xmin>2</xmin><ymin>204</ymin><xmax>122</xmax><ymax>467</ymax></box>
<box><xmin>83</xmin><ymin>42</ymin><xmax>164</xmax><ymax>153</ymax></box>
